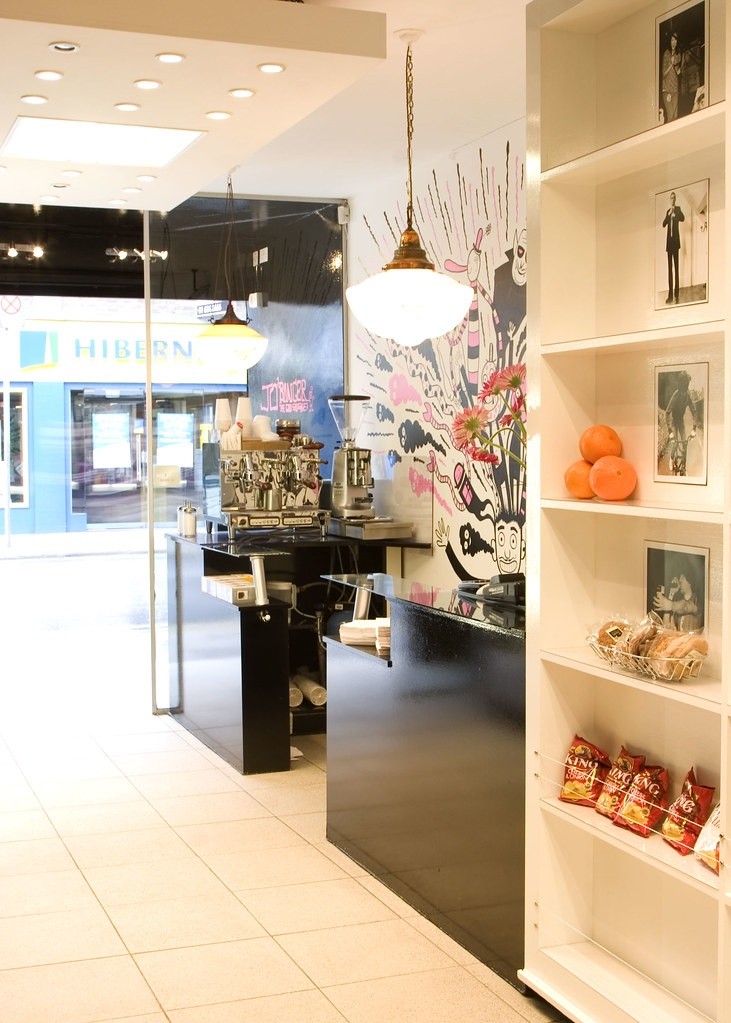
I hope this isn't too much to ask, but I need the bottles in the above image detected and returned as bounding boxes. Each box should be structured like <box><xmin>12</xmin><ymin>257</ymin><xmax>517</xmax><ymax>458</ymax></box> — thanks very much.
<box><xmin>184</xmin><ymin>501</ymin><xmax>197</xmax><ymax>538</ymax></box>
<box><xmin>177</xmin><ymin>500</ymin><xmax>187</xmax><ymax>536</ymax></box>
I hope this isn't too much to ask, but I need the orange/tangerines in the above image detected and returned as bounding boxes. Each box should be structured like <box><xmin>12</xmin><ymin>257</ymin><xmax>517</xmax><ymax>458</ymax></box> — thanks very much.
<box><xmin>565</xmin><ymin>426</ymin><xmax>638</xmax><ymax>500</ymax></box>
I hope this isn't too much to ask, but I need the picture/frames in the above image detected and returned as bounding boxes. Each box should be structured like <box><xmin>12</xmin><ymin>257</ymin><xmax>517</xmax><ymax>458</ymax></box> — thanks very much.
<box><xmin>654</xmin><ymin>360</ymin><xmax>709</xmax><ymax>486</ymax></box>
<box><xmin>655</xmin><ymin>1</ymin><xmax>710</xmax><ymax>127</ymax></box>
<box><xmin>655</xmin><ymin>177</ymin><xmax>710</xmax><ymax>311</ymax></box>
<box><xmin>642</xmin><ymin>539</ymin><xmax>711</xmax><ymax>637</ymax></box>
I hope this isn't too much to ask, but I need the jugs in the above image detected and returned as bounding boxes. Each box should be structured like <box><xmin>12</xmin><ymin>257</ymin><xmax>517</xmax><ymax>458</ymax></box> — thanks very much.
<box><xmin>267</xmin><ymin>581</ymin><xmax>296</xmax><ymax>625</ymax></box>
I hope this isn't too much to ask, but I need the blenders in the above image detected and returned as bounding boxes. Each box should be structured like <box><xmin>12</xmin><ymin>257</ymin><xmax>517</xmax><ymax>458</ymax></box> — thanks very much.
<box><xmin>328</xmin><ymin>394</ymin><xmax>375</xmax><ymax>519</ymax></box>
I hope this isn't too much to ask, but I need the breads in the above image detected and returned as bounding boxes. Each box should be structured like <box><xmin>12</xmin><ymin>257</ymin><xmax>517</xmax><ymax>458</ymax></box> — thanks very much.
<box><xmin>595</xmin><ymin>620</ymin><xmax>709</xmax><ymax>682</ymax></box>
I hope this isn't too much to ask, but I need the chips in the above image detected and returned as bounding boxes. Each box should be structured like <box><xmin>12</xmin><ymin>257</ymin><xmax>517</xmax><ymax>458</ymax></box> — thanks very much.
<box><xmin>559</xmin><ymin>780</ymin><xmax>685</xmax><ymax>842</ymax></box>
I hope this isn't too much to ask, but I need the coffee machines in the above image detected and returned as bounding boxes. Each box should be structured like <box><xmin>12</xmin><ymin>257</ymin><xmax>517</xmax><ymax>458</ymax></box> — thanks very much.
<box><xmin>201</xmin><ymin>442</ymin><xmax>331</xmax><ymax>542</ymax></box>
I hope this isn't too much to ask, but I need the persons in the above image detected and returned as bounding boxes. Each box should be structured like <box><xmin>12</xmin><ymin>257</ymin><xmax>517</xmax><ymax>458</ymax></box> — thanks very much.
<box><xmin>664</xmin><ymin>369</ymin><xmax>698</xmax><ymax>476</ymax></box>
<box><xmin>662</xmin><ymin>191</ymin><xmax>685</xmax><ymax>305</ymax></box>
<box><xmin>662</xmin><ymin>32</ymin><xmax>681</xmax><ymax>123</ymax></box>
<box><xmin>652</xmin><ymin>568</ymin><xmax>702</xmax><ymax>636</ymax></box>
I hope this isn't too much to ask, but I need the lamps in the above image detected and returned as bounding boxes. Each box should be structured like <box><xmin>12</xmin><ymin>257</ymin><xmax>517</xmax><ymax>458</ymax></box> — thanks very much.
<box><xmin>112</xmin><ymin>246</ymin><xmax>127</xmax><ymax>260</ymax></box>
<box><xmin>197</xmin><ymin>173</ymin><xmax>270</xmax><ymax>372</ymax></box>
<box><xmin>33</xmin><ymin>242</ymin><xmax>44</xmax><ymax>258</ymax></box>
<box><xmin>8</xmin><ymin>236</ymin><xmax>18</xmax><ymax>257</ymax></box>
<box><xmin>346</xmin><ymin>28</ymin><xmax>475</xmax><ymax>346</ymax></box>
<box><xmin>134</xmin><ymin>247</ymin><xmax>146</xmax><ymax>260</ymax></box>
<box><xmin>152</xmin><ymin>249</ymin><xmax>169</xmax><ymax>260</ymax></box>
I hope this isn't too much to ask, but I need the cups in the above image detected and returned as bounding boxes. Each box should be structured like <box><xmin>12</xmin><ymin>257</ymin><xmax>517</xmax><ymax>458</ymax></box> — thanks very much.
<box><xmin>263</xmin><ymin>490</ymin><xmax>287</xmax><ymax>511</ymax></box>
<box><xmin>214</xmin><ymin>396</ymin><xmax>254</xmax><ymax>441</ymax></box>
<box><xmin>288</xmin><ymin>674</ymin><xmax>327</xmax><ymax>708</ymax></box>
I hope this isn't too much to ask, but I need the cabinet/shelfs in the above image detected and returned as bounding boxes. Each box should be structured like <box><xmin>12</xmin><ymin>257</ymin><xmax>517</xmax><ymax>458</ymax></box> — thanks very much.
<box><xmin>519</xmin><ymin>0</ymin><xmax>731</xmax><ymax>1023</ymax></box>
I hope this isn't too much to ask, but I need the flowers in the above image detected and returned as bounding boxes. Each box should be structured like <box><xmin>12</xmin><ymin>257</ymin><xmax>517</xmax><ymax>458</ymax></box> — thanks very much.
<box><xmin>453</xmin><ymin>364</ymin><xmax>526</xmax><ymax>471</ymax></box>
